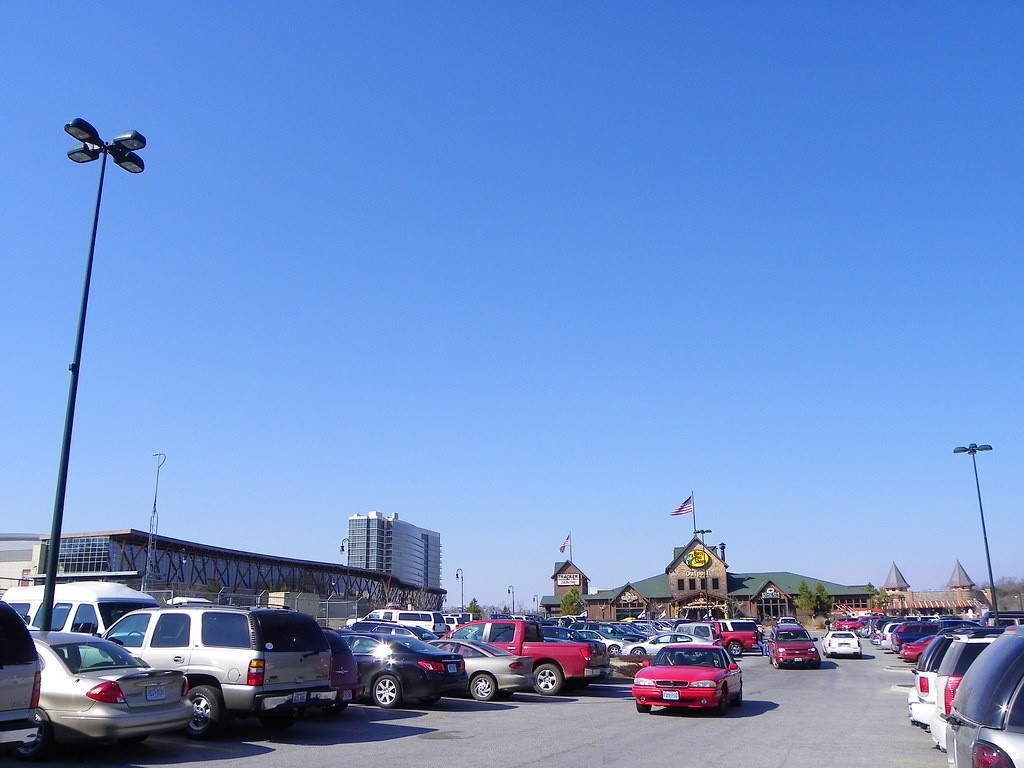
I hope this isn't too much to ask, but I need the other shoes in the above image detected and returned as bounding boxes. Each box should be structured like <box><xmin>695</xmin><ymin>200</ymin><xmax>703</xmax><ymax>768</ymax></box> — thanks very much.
<box><xmin>763</xmin><ymin>655</ymin><xmax>769</xmax><ymax>657</ymax></box>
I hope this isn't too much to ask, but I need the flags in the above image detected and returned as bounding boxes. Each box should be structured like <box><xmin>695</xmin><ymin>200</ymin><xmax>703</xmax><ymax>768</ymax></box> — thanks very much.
<box><xmin>559</xmin><ymin>535</ymin><xmax>570</xmax><ymax>554</ymax></box>
<box><xmin>669</xmin><ymin>496</ymin><xmax>693</xmax><ymax>515</ymax></box>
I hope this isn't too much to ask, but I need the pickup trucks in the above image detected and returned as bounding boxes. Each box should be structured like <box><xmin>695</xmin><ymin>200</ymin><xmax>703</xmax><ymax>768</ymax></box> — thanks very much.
<box><xmin>424</xmin><ymin>619</ymin><xmax>611</xmax><ymax>697</ymax></box>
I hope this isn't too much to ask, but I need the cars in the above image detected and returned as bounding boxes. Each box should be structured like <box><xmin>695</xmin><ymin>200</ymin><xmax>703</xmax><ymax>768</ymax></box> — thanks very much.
<box><xmin>1</xmin><ymin>581</ymin><xmax>161</xmax><ymax>637</ymax></box>
<box><xmin>12</xmin><ymin>631</ymin><xmax>194</xmax><ymax>763</ymax></box>
<box><xmin>0</xmin><ymin>600</ymin><xmax>42</xmax><ymax>760</ymax></box>
<box><xmin>820</xmin><ymin>630</ymin><xmax>862</xmax><ymax>658</ymax></box>
<box><xmin>940</xmin><ymin>625</ymin><xmax>1024</xmax><ymax>768</ymax></box>
<box><xmin>343</xmin><ymin>634</ymin><xmax>469</xmax><ymax>710</ymax></box>
<box><xmin>427</xmin><ymin>640</ymin><xmax>536</xmax><ymax>701</ymax></box>
<box><xmin>294</xmin><ymin>627</ymin><xmax>367</xmax><ymax>721</ymax></box>
<box><xmin>632</xmin><ymin>644</ymin><xmax>743</xmax><ymax>717</ymax></box>
<box><xmin>336</xmin><ymin>608</ymin><xmax>764</xmax><ymax>663</ymax></box>
<box><xmin>776</xmin><ymin>616</ymin><xmax>801</xmax><ymax>625</ymax></box>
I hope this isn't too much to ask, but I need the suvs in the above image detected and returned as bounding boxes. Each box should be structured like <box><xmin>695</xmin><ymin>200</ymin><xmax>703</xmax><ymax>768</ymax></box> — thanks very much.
<box><xmin>907</xmin><ymin>610</ymin><xmax>1024</xmax><ymax>751</ymax></box>
<box><xmin>766</xmin><ymin>624</ymin><xmax>821</xmax><ymax>668</ymax></box>
<box><xmin>835</xmin><ymin>616</ymin><xmax>980</xmax><ymax>669</ymax></box>
<box><xmin>76</xmin><ymin>602</ymin><xmax>337</xmax><ymax>742</ymax></box>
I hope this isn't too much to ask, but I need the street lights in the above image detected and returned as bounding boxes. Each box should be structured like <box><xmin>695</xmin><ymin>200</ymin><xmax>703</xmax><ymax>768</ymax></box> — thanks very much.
<box><xmin>39</xmin><ymin>117</ymin><xmax>148</xmax><ymax>632</ymax></box>
<box><xmin>339</xmin><ymin>538</ymin><xmax>350</xmax><ymax>601</ymax></box>
<box><xmin>455</xmin><ymin>568</ymin><xmax>463</xmax><ymax>613</ymax></box>
<box><xmin>953</xmin><ymin>443</ymin><xmax>1001</xmax><ymax>627</ymax></box>
<box><xmin>693</xmin><ymin>529</ymin><xmax>713</xmax><ymax>621</ymax></box>
<box><xmin>507</xmin><ymin>585</ymin><xmax>539</xmax><ymax>618</ymax></box>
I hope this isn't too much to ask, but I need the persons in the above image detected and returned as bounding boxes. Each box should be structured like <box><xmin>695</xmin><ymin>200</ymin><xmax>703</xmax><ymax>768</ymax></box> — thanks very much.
<box><xmin>824</xmin><ymin>618</ymin><xmax>831</xmax><ymax>634</ymax></box>
<box><xmin>756</xmin><ymin>627</ymin><xmax>768</xmax><ymax>658</ymax></box>
